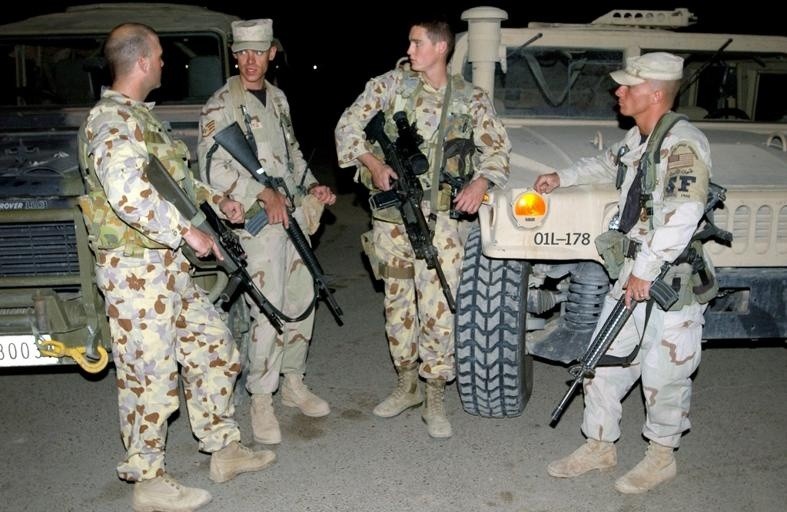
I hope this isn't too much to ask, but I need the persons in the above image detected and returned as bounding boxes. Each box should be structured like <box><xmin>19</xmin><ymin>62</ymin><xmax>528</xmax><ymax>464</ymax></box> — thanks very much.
<box><xmin>535</xmin><ymin>53</ymin><xmax>716</xmax><ymax>495</ymax></box>
<box><xmin>198</xmin><ymin>17</ymin><xmax>343</xmax><ymax>445</ymax></box>
<box><xmin>334</xmin><ymin>20</ymin><xmax>510</xmax><ymax>438</ymax></box>
<box><xmin>79</xmin><ymin>23</ymin><xmax>276</xmax><ymax>512</ymax></box>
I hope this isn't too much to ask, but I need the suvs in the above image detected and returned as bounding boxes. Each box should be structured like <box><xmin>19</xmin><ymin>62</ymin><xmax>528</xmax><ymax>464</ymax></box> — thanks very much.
<box><xmin>443</xmin><ymin>5</ymin><xmax>787</xmax><ymax>417</ymax></box>
<box><xmin>0</xmin><ymin>4</ymin><xmax>282</xmax><ymax>370</ymax></box>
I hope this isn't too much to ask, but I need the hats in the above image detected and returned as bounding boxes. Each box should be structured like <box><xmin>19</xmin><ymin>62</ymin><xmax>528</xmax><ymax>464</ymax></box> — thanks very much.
<box><xmin>231</xmin><ymin>18</ymin><xmax>273</xmax><ymax>53</ymax></box>
<box><xmin>609</xmin><ymin>52</ymin><xmax>684</xmax><ymax>86</ymax></box>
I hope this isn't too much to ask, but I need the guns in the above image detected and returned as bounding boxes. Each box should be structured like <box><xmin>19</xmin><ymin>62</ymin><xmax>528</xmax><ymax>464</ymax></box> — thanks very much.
<box><xmin>361</xmin><ymin>108</ymin><xmax>458</xmax><ymax>313</ymax></box>
<box><xmin>548</xmin><ymin>184</ymin><xmax>734</xmax><ymax>422</ymax></box>
<box><xmin>207</xmin><ymin>123</ymin><xmax>345</xmax><ymax>318</ymax></box>
<box><xmin>143</xmin><ymin>154</ymin><xmax>283</xmax><ymax>328</ymax></box>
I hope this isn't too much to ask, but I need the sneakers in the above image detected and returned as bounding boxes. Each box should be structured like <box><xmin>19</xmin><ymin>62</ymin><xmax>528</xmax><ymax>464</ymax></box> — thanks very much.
<box><xmin>614</xmin><ymin>440</ymin><xmax>677</xmax><ymax>494</ymax></box>
<box><xmin>422</xmin><ymin>378</ymin><xmax>453</xmax><ymax>439</ymax></box>
<box><xmin>133</xmin><ymin>473</ymin><xmax>212</xmax><ymax>512</ymax></box>
<box><xmin>209</xmin><ymin>441</ymin><xmax>277</xmax><ymax>483</ymax></box>
<box><xmin>281</xmin><ymin>371</ymin><xmax>330</xmax><ymax>417</ymax></box>
<box><xmin>372</xmin><ymin>362</ymin><xmax>424</xmax><ymax>418</ymax></box>
<box><xmin>250</xmin><ymin>393</ymin><xmax>282</xmax><ymax>445</ymax></box>
<box><xmin>547</xmin><ymin>438</ymin><xmax>617</xmax><ymax>478</ymax></box>
